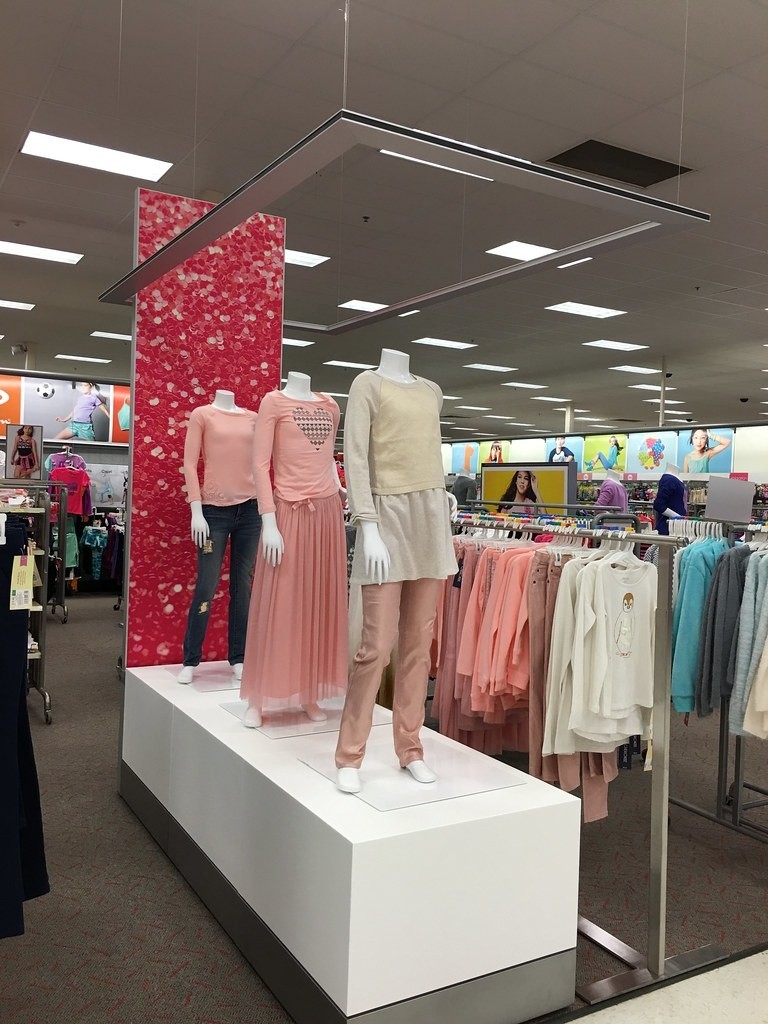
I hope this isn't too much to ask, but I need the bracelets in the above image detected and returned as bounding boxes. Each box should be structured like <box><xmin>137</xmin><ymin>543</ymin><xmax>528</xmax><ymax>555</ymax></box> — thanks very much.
<box><xmin>713</xmin><ymin>435</ymin><xmax>715</xmax><ymax>441</ymax></box>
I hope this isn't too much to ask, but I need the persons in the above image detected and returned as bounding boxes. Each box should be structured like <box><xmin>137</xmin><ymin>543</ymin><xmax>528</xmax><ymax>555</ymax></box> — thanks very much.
<box><xmin>54</xmin><ymin>382</ymin><xmax>110</xmax><ymax>442</ymax></box>
<box><xmin>336</xmin><ymin>347</ymin><xmax>458</xmax><ymax>793</ymax></box>
<box><xmin>548</xmin><ymin>437</ymin><xmax>574</xmax><ymax>462</ymax></box>
<box><xmin>177</xmin><ymin>390</ymin><xmax>257</xmax><ymax>685</ymax></box>
<box><xmin>497</xmin><ymin>472</ymin><xmax>547</xmax><ymax>514</ymax></box>
<box><xmin>594</xmin><ymin>468</ymin><xmax>628</xmax><ymax>514</ymax></box>
<box><xmin>653</xmin><ymin>462</ymin><xmax>686</xmax><ymax>536</ymax></box>
<box><xmin>485</xmin><ymin>441</ymin><xmax>506</xmax><ymax>463</ymax></box>
<box><xmin>239</xmin><ymin>371</ymin><xmax>349</xmax><ymax>726</ymax></box>
<box><xmin>684</xmin><ymin>428</ymin><xmax>731</xmax><ymax>473</ymax></box>
<box><xmin>586</xmin><ymin>436</ymin><xmax>623</xmax><ymax>471</ymax></box>
<box><xmin>10</xmin><ymin>426</ymin><xmax>39</xmax><ymax>478</ymax></box>
<box><xmin>453</xmin><ymin>468</ymin><xmax>477</xmax><ymax>504</ymax></box>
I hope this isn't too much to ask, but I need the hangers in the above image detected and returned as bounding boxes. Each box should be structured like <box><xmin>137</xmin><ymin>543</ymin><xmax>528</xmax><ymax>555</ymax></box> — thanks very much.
<box><xmin>58</xmin><ymin>445</ymin><xmax>75</xmax><ymax>456</ymax></box>
<box><xmin>62</xmin><ymin>459</ymin><xmax>78</xmax><ymax>471</ymax></box>
<box><xmin>83</xmin><ymin>507</ymin><xmax>127</xmax><ymax>536</ymax></box>
<box><xmin>452</xmin><ymin>505</ymin><xmax>635</xmax><ymax>567</ymax></box>
<box><xmin>666</xmin><ymin>519</ymin><xmax>768</xmax><ymax>558</ymax></box>
<box><xmin>340</xmin><ymin>508</ymin><xmax>356</xmax><ymax>526</ymax></box>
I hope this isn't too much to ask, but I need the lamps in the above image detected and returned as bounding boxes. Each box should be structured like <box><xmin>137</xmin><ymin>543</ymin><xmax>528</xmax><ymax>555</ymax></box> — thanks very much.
<box><xmin>11</xmin><ymin>342</ymin><xmax>28</xmax><ymax>356</ymax></box>
